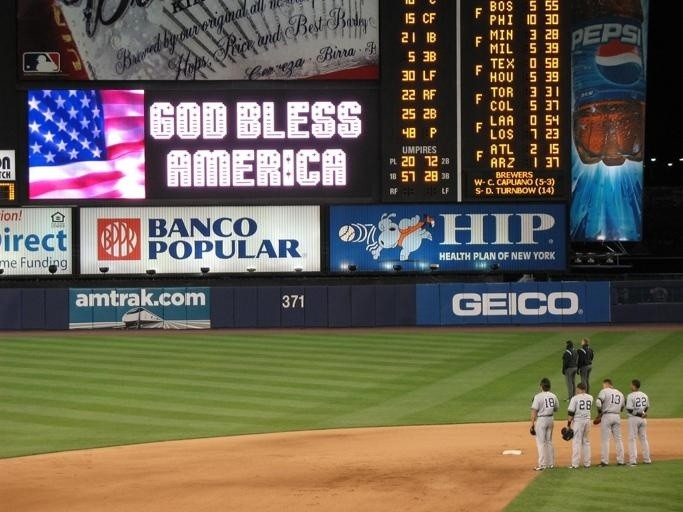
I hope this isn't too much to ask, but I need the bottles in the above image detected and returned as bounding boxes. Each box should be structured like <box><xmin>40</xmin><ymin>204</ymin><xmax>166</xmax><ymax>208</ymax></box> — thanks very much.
<box><xmin>571</xmin><ymin>0</ymin><xmax>646</xmax><ymax>167</ymax></box>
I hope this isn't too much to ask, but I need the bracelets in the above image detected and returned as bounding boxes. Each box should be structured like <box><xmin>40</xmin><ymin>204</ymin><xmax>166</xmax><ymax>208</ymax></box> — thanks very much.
<box><xmin>567</xmin><ymin>420</ymin><xmax>571</xmax><ymax>427</ymax></box>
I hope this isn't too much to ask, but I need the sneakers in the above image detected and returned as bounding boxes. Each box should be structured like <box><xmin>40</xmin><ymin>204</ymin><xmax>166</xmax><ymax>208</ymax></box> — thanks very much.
<box><xmin>569</xmin><ymin>465</ymin><xmax>590</xmax><ymax>470</ymax></box>
<box><xmin>601</xmin><ymin>462</ymin><xmax>608</xmax><ymax>466</ymax></box>
<box><xmin>617</xmin><ymin>461</ymin><xmax>652</xmax><ymax>465</ymax></box>
<box><xmin>534</xmin><ymin>465</ymin><xmax>555</xmax><ymax>471</ymax></box>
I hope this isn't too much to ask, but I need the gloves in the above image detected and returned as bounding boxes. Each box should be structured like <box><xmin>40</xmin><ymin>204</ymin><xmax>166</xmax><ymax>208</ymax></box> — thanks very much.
<box><xmin>642</xmin><ymin>413</ymin><xmax>647</xmax><ymax>418</ymax></box>
<box><xmin>530</xmin><ymin>427</ymin><xmax>536</xmax><ymax>436</ymax></box>
<box><xmin>594</xmin><ymin>418</ymin><xmax>601</xmax><ymax>425</ymax></box>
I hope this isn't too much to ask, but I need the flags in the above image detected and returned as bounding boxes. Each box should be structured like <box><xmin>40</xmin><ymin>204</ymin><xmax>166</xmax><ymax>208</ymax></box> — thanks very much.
<box><xmin>25</xmin><ymin>88</ymin><xmax>147</xmax><ymax>200</ymax></box>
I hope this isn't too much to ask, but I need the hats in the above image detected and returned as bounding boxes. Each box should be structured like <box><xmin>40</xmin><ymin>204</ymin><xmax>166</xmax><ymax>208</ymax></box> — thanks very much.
<box><xmin>563</xmin><ymin>429</ymin><xmax>573</xmax><ymax>440</ymax></box>
<box><xmin>540</xmin><ymin>378</ymin><xmax>551</xmax><ymax>386</ymax></box>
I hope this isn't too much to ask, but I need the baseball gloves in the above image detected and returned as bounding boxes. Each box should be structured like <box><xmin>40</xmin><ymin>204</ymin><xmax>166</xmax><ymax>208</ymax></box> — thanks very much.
<box><xmin>530</xmin><ymin>427</ymin><xmax>536</xmax><ymax>435</ymax></box>
<box><xmin>593</xmin><ymin>417</ymin><xmax>600</xmax><ymax>424</ymax></box>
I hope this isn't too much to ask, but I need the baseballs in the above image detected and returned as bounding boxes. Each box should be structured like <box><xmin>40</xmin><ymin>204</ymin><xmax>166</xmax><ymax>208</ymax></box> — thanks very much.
<box><xmin>26</xmin><ymin>66</ymin><xmax>30</xmax><ymax>68</ymax></box>
<box><xmin>339</xmin><ymin>226</ymin><xmax>355</xmax><ymax>242</ymax></box>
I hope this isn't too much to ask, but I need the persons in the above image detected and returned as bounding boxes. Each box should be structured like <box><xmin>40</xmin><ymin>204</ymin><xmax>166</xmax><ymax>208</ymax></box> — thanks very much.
<box><xmin>529</xmin><ymin>377</ymin><xmax>559</xmax><ymax>470</ymax></box>
<box><xmin>595</xmin><ymin>378</ymin><xmax>627</xmax><ymax>467</ymax></box>
<box><xmin>625</xmin><ymin>379</ymin><xmax>652</xmax><ymax>466</ymax></box>
<box><xmin>566</xmin><ymin>382</ymin><xmax>594</xmax><ymax>469</ymax></box>
<box><xmin>562</xmin><ymin>340</ymin><xmax>578</xmax><ymax>402</ymax></box>
<box><xmin>576</xmin><ymin>338</ymin><xmax>594</xmax><ymax>394</ymax></box>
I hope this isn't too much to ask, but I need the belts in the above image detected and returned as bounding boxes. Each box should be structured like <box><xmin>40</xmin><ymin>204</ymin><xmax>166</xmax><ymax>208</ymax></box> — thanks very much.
<box><xmin>603</xmin><ymin>412</ymin><xmax>618</xmax><ymax>414</ymax></box>
<box><xmin>538</xmin><ymin>415</ymin><xmax>553</xmax><ymax>417</ymax></box>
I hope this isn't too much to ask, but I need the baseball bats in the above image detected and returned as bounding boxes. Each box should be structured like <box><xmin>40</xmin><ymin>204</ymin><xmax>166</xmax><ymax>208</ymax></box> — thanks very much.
<box><xmin>46</xmin><ymin>54</ymin><xmax>55</xmax><ymax>64</ymax></box>
